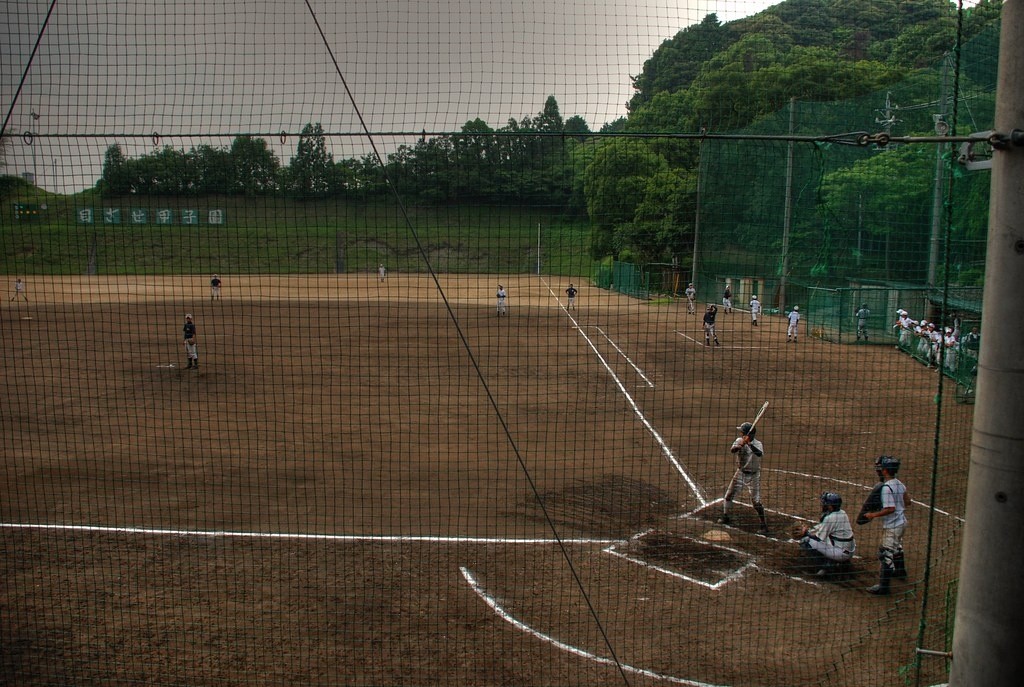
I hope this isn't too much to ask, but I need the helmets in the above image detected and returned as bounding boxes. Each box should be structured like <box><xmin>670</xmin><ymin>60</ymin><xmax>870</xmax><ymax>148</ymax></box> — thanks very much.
<box><xmin>737</xmin><ymin>422</ymin><xmax>757</xmax><ymax>439</ymax></box>
<box><xmin>875</xmin><ymin>455</ymin><xmax>900</xmax><ymax>473</ymax></box>
<box><xmin>820</xmin><ymin>493</ymin><xmax>842</xmax><ymax>507</ymax></box>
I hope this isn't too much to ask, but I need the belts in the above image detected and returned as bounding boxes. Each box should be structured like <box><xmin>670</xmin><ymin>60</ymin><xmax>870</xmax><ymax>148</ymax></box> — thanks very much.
<box><xmin>738</xmin><ymin>467</ymin><xmax>756</xmax><ymax>475</ymax></box>
<box><xmin>843</xmin><ymin>550</ymin><xmax>850</xmax><ymax>554</ymax></box>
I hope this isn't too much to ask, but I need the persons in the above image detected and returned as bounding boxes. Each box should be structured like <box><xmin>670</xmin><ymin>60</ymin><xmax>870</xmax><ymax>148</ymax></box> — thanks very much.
<box><xmin>791</xmin><ymin>492</ymin><xmax>855</xmax><ymax>576</ymax></box>
<box><xmin>378</xmin><ymin>264</ymin><xmax>386</xmax><ymax>282</ymax></box>
<box><xmin>718</xmin><ymin>423</ymin><xmax>767</xmax><ymax>534</ymax></box>
<box><xmin>787</xmin><ymin>305</ymin><xmax>800</xmax><ymax>343</ymax></box>
<box><xmin>896</xmin><ymin>309</ymin><xmax>956</xmax><ymax>375</ymax></box>
<box><xmin>952</xmin><ymin>313</ymin><xmax>961</xmax><ymax>340</ymax></box>
<box><xmin>497</xmin><ymin>285</ymin><xmax>506</xmax><ymax>317</ymax></box>
<box><xmin>183</xmin><ymin>314</ymin><xmax>199</xmax><ymax>370</ymax></box>
<box><xmin>11</xmin><ymin>279</ymin><xmax>27</xmax><ymax>301</ymax></box>
<box><xmin>966</xmin><ymin>326</ymin><xmax>981</xmax><ymax>357</ymax></box>
<box><xmin>211</xmin><ymin>273</ymin><xmax>221</xmax><ymax>300</ymax></box>
<box><xmin>856</xmin><ymin>303</ymin><xmax>870</xmax><ymax>341</ymax></box>
<box><xmin>702</xmin><ymin>304</ymin><xmax>719</xmax><ymax>346</ymax></box>
<box><xmin>685</xmin><ymin>282</ymin><xmax>695</xmax><ymax>314</ymax></box>
<box><xmin>864</xmin><ymin>456</ymin><xmax>911</xmax><ymax>596</ymax></box>
<box><xmin>750</xmin><ymin>295</ymin><xmax>760</xmax><ymax>326</ymax></box>
<box><xmin>566</xmin><ymin>284</ymin><xmax>577</xmax><ymax>310</ymax></box>
<box><xmin>723</xmin><ymin>285</ymin><xmax>732</xmax><ymax>314</ymax></box>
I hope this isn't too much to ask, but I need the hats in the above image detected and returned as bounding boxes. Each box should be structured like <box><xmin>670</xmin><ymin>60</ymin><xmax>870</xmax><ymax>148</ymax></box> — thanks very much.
<box><xmin>862</xmin><ymin>303</ymin><xmax>867</xmax><ymax>307</ymax></box>
<box><xmin>689</xmin><ymin>283</ymin><xmax>693</xmax><ymax>286</ymax></box>
<box><xmin>794</xmin><ymin>305</ymin><xmax>799</xmax><ymax>310</ymax></box>
<box><xmin>896</xmin><ymin>309</ymin><xmax>953</xmax><ymax>334</ymax></box>
<box><xmin>751</xmin><ymin>295</ymin><xmax>757</xmax><ymax>299</ymax></box>
<box><xmin>186</xmin><ymin>313</ymin><xmax>192</xmax><ymax>318</ymax></box>
<box><xmin>707</xmin><ymin>306</ymin><xmax>713</xmax><ymax>310</ymax></box>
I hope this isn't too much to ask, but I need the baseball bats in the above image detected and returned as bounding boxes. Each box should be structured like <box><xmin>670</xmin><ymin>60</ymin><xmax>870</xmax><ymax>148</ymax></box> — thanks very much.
<box><xmin>741</xmin><ymin>401</ymin><xmax>770</xmax><ymax>447</ymax></box>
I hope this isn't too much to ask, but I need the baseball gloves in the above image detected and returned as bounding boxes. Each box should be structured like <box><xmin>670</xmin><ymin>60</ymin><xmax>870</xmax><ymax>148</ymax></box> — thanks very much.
<box><xmin>188</xmin><ymin>337</ymin><xmax>196</xmax><ymax>345</ymax></box>
<box><xmin>791</xmin><ymin>520</ymin><xmax>811</xmax><ymax>540</ymax></box>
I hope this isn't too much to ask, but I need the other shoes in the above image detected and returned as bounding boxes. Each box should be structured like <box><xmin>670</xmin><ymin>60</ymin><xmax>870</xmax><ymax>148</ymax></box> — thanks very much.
<box><xmin>717</xmin><ymin>515</ymin><xmax>733</xmax><ymax>524</ymax></box>
<box><xmin>816</xmin><ymin>563</ymin><xmax>834</xmax><ymax>577</ymax></box>
<box><xmin>866</xmin><ymin>584</ymin><xmax>889</xmax><ymax>595</ymax></box>
<box><xmin>756</xmin><ymin>525</ymin><xmax>768</xmax><ymax>534</ymax></box>
<box><xmin>893</xmin><ymin>569</ymin><xmax>906</xmax><ymax>581</ymax></box>
